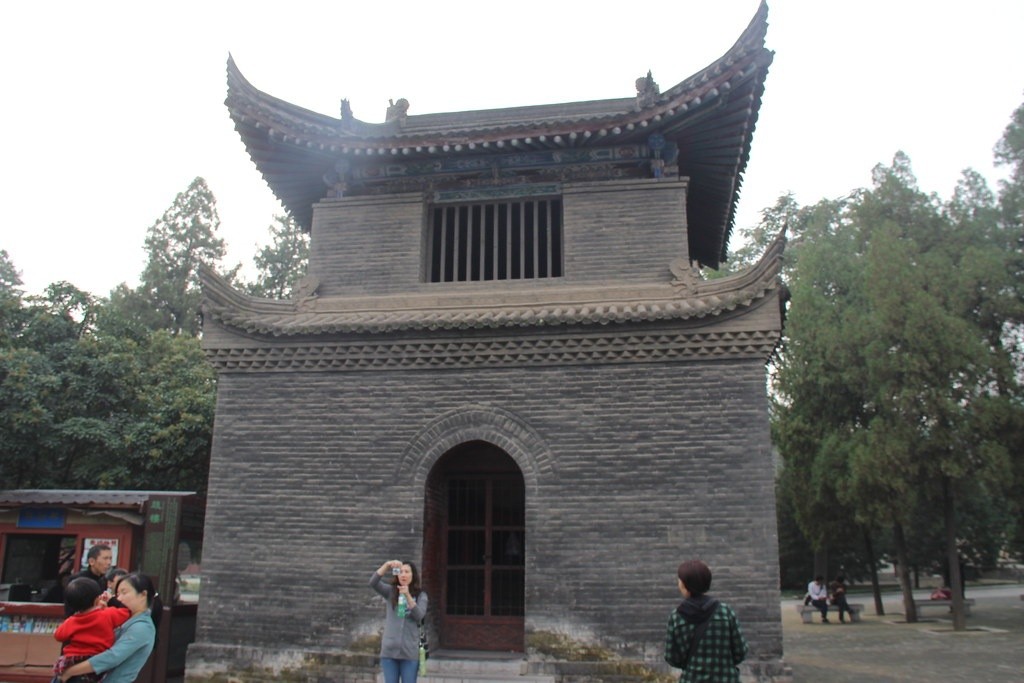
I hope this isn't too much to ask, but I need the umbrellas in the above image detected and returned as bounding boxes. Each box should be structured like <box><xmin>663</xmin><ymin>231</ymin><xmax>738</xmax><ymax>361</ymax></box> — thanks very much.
<box><xmin>418</xmin><ymin>643</ymin><xmax>427</xmax><ymax>677</ymax></box>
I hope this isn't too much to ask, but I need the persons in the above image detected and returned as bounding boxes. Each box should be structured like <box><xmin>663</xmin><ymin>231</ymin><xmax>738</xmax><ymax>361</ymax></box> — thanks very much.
<box><xmin>831</xmin><ymin>576</ymin><xmax>854</xmax><ymax>624</ymax></box>
<box><xmin>369</xmin><ymin>560</ymin><xmax>429</xmax><ymax>683</ymax></box>
<box><xmin>663</xmin><ymin>560</ymin><xmax>749</xmax><ymax>683</ymax></box>
<box><xmin>106</xmin><ymin>568</ymin><xmax>129</xmax><ymax>596</ymax></box>
<box><xmin>60</xmin><ymin>544</ymin><xmax>113</xmax><ymax>657</ymax></box>
<box><xmin>938</xmin><ymin>537</ymin><xmax>970</xmax><ymax>613</ymax></box>
<box><xmin>808</xmin><ymin>575</ymin><xmax>829</xmax><ymax>624</ymax></box>
<box><xmin>55</xmin><ymin>571</ymin><xmax>163</xmax><ymax>683</ymax></box>
<box><xmin>53</xmin><ymin>577</ymin><xmax>133</xmax><ymax>683</ymax></box>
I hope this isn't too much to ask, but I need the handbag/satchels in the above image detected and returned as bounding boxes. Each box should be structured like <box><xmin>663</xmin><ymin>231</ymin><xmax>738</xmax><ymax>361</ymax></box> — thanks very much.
<box><xmin>803</xmin><ymin>594</ymin><xmax>810</xmax><ymax>605</ymax></box>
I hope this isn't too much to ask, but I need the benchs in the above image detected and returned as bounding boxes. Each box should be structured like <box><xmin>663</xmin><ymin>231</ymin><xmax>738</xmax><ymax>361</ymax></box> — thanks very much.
<box><xmin>903</xmin><ymin>598</ymin><xmax>975</xmax><ymax>618</ymax></box>
<box><xmin>797</xmin><ymin>603</ymin><xmax>864</xmax><ymax>624</ymax></box>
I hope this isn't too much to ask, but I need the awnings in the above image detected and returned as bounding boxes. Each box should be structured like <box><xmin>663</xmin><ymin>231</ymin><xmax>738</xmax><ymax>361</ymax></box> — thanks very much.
<box><xmin>0</xmin><ymin>489</ymin><xmax>148</xmax><ymax>514</ymax></box>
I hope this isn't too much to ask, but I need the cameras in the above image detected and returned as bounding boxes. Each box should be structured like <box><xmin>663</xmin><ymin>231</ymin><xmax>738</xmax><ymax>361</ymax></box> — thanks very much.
<box><xmin>393</xmin><ymin>568</ymin><xmax>401</xmax><ymax>575</ymax></box>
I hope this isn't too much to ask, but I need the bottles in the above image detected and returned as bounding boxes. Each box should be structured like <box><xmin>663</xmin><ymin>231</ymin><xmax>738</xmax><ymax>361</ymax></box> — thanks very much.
<box><xmin>397</xmin><ymin>593</ymin><xmax>406</xmax><ymax>617</ymax></box>
